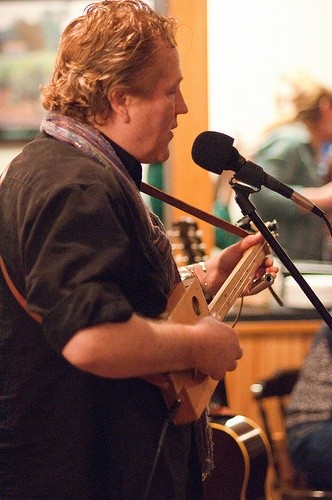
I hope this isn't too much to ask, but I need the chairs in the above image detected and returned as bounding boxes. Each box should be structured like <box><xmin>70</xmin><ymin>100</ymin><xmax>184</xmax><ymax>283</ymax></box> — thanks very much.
<box><xmin>251</xmin><ymin>369</ymin><xmax>330</xmax><ymax>499</ymax></box>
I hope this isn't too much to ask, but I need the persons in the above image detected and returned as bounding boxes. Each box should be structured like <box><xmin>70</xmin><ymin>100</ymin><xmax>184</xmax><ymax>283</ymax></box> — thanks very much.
<box><xmin>0</xmin><ymin>0</ymin><xmax>278</xmax><ymax>500</ymax></box>
<box><xmin>234</xmin><ymin>78</ymin><xmax>331</xmax><ymax>309</ymax></box>
<box><xmin>283</xmin><ymin>310</ymin><xmax>332</xmax><ymax>491</ymax></box>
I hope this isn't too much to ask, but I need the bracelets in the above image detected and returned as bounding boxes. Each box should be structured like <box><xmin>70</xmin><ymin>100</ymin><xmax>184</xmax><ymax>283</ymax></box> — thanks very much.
<box><xmin>185</xmin><ymin>263</ymin><xmax>198</xmax><ymax>279</ymax></box>
<box><xmin>199</xmin><ymin>261</ymin><xmax>210</xmax><ymax>300</ymax></box>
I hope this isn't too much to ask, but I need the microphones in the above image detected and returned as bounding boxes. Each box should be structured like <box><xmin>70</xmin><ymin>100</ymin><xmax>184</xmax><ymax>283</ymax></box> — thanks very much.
<box><xmin>192</xmin><ymin>131</ymin><xmax>325</xmax><ymax>217</ymax></box>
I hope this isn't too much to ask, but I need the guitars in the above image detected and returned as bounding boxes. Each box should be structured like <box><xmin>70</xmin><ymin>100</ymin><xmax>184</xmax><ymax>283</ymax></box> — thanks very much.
<box><xmin>134</xmin><ymin>210</ymin><xmax>278</xmax><ymax>428</ymax></box>
<box><xmin>166</xmin><ymin>215</ymin><xmax>274</xmax><ymax>500</ymax></box>
